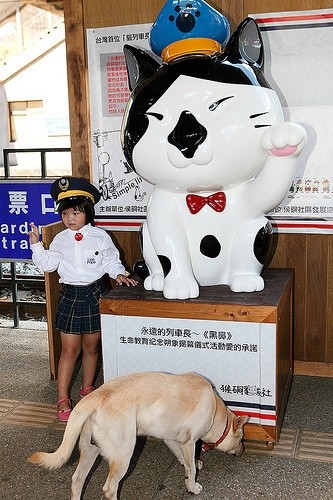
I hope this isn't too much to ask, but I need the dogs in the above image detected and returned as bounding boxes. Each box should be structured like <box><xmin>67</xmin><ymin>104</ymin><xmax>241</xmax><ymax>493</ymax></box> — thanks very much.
<box><xmin>27</xmin><ymin>371</ymin><xmax>249</xmax><ymax>500</ymax></box>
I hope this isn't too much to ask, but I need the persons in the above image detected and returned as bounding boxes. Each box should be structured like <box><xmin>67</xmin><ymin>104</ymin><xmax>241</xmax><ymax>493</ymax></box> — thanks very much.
<box><xmin>28</xmin><ymin>177</ymin><xmax>139</xmax><ymax>425</ymax></box>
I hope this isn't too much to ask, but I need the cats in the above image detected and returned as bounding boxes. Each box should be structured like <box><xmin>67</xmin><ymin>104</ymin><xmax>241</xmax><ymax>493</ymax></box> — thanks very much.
<box><xmin>123</xmin><ymin>17</ymin><xmax>307</xmax><ymax>299</ymax></box>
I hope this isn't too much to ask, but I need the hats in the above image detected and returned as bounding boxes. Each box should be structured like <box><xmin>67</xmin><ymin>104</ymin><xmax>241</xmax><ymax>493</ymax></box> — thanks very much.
<box><xmin>50</xmin><ymin>176</ymin><xmax>101</xmax><ymax>212</ymax></box>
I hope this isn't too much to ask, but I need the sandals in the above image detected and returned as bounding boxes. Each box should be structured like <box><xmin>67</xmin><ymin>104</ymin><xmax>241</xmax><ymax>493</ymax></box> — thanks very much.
<box><xmin>57</xmin><ymin>398</ymin><xmax>71</xmax><ymax>421</ymax></box>
<box><xmin>79</xmin><ymin>385</ymin><xmax>94</xmax><ymax>397</ymax></box>
